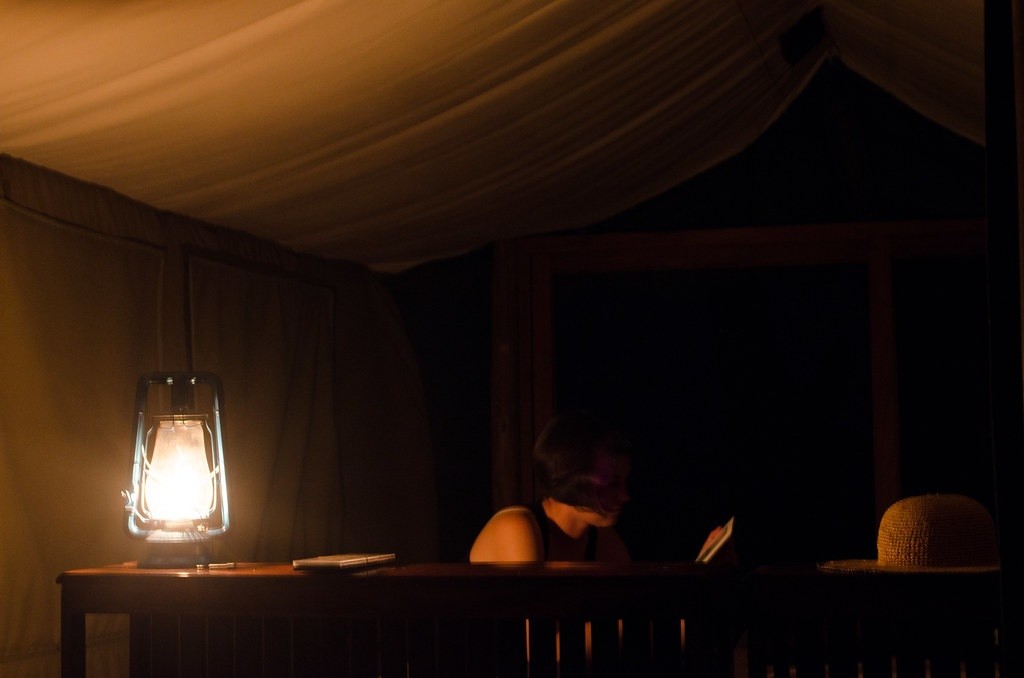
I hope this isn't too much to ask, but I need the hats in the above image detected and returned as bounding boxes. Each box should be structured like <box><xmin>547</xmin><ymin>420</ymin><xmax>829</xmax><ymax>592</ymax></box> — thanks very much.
<box><xmin>815</xmin><ymin>494</ymin><xmax>1001</xmax><ymax>575</ymax></box>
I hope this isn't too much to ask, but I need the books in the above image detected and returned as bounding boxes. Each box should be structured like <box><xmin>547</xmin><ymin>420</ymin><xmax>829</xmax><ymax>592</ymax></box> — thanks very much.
<box><xmin>292</xmin><ymin>549</ymin><xmax>399</xmax><ymax>576</ymax></box>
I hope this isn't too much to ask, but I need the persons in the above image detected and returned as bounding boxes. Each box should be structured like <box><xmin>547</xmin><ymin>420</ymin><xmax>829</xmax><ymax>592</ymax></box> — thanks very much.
<box><xmin>466</xmin><ymin>396</ymin><xmax>725</xmax><ymax>563</ymax></box>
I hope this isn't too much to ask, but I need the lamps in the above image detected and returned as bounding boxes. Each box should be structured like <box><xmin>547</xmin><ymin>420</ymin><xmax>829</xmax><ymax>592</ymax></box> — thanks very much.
<box><xmin>120</xmin><ymin>369</ymin><xmax>230</xmax><ymax>568</ymax></box>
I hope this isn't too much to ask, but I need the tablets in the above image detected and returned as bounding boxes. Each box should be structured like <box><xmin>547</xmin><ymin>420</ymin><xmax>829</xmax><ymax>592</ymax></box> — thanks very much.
<box><xmin>697</xmin><ymin>519</ymin><xmax>734</xmax><ymax>562</ymax></box>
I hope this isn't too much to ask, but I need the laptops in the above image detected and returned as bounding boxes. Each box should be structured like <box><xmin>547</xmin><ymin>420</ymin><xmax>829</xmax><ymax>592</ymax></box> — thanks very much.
<box><xmin>292</xmin><ymin>553</ymin><xmax>395</xmax><ymax>570</ymax></box>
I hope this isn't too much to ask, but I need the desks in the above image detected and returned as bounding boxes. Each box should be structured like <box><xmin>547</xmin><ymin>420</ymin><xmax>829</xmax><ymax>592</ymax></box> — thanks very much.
<box><xmin>55</xmin><ymin>563</ymin><xmax>738</xmax><ymax>678</ymax></box>
<box><xmin>745</xmin><ymin>564</ymin><xmax>1024</xmax><ymax>678</ymax></box>
